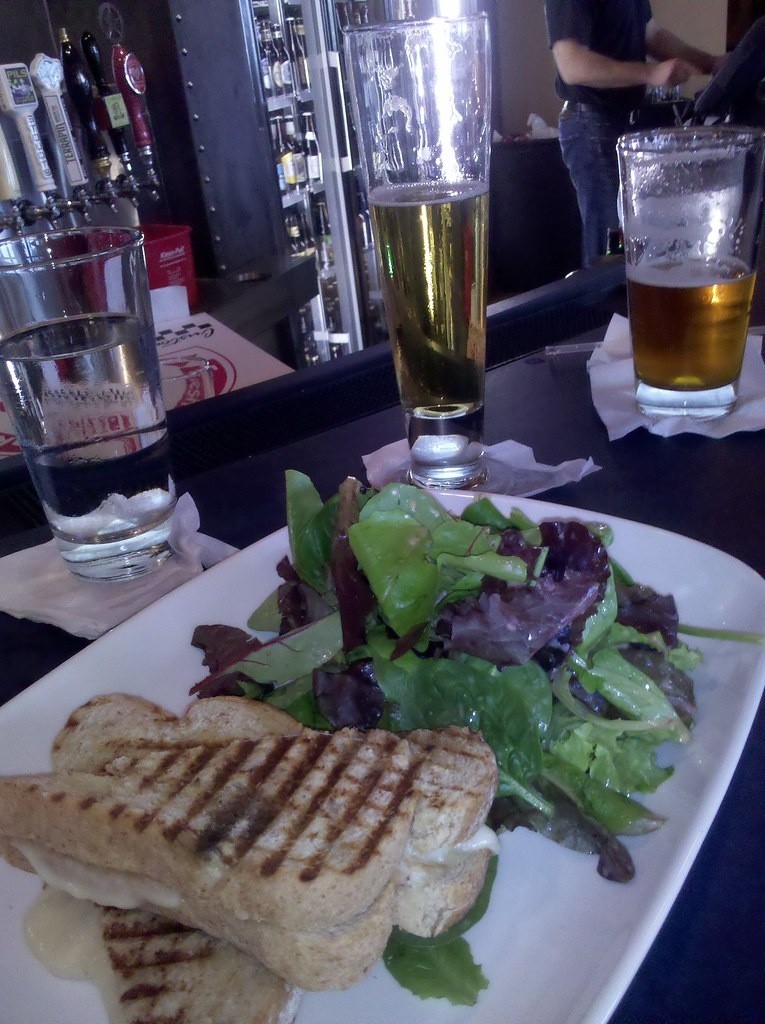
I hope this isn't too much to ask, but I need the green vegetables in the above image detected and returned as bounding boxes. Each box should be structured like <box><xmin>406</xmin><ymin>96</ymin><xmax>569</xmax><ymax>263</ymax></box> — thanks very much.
<box><xmin>186</xmin><ymin>469</ymin><xmax>765</xmax><ymax>1008</ymax></box>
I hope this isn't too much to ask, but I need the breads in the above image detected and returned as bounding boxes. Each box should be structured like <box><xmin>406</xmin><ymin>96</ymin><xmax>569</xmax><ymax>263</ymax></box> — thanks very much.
<box><xmin>0</xmin><ymin>690</ymin><xmax>497</xmax><ymax>1024</ymax></box>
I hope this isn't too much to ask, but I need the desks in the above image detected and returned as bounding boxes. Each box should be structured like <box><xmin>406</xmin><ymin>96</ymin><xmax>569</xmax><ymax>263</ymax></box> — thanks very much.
<box><xmin>642</xmin><ymin>95</ymin><xmax>695</xmax><ymax>130</ymax></box>
<box><xmin>0</xmin><ymin>312</ymin><xmax>296</xmax><ymax>460</ymax></box>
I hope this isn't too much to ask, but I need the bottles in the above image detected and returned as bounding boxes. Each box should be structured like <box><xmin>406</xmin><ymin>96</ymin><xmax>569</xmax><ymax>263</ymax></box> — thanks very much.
<box><xmin>250</xmin><ymin>13</ymin><xmax>391</xmax><ymax>366</ymax></box>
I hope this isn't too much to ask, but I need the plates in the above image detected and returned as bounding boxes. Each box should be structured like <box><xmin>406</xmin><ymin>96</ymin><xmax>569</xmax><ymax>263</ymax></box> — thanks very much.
<box><xmin>0</xmin><ymin>486</ymin><xmax>764</xmax><ymax>1024</ymax></box>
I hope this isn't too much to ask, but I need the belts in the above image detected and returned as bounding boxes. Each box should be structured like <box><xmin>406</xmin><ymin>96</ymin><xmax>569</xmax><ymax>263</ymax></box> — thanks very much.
<box><xmin>563</xmin><ymin>100</ymin><xmax>640</xmax><ymax>125</ymax></box>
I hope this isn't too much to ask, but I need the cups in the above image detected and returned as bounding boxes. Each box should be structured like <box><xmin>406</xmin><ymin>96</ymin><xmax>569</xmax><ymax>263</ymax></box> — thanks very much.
<box><xmin>615</xmin><ymin>124</ymin><xmax>765</xmax><ymax>426</ymax></box>
<box><xmin>0</xmin><ymin>225</ymin><xmax>179</xmax><ymax>582</ymax></box>
<box><xmin>332</xmin><ymin>0</ymin><xmax>493</xmax><ymax>491</ymax></box>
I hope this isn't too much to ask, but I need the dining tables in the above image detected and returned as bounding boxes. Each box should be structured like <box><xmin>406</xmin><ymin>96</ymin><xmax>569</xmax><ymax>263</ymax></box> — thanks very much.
<box><xmin>0</xmin><ymin>256</ymin><xmax>765</xmax><ymax>1024</ymax></box>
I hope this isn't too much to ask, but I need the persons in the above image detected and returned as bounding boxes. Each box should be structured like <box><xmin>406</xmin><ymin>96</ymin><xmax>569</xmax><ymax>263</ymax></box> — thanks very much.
<box><xmin>544</xmin><ymin>0</ymin><xmax>730</xmax><ymax>269</ymax></box>
